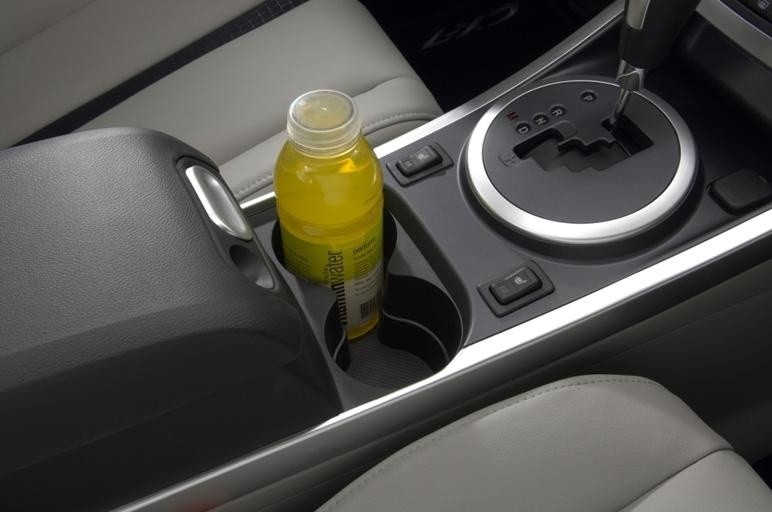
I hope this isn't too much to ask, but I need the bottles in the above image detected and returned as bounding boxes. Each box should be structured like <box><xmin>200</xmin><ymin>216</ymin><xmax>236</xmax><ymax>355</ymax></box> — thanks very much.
<box><xmin>272</xmin><ymin>86</ymin><xmax>385</xmax><ymax>338</ymax></box>
